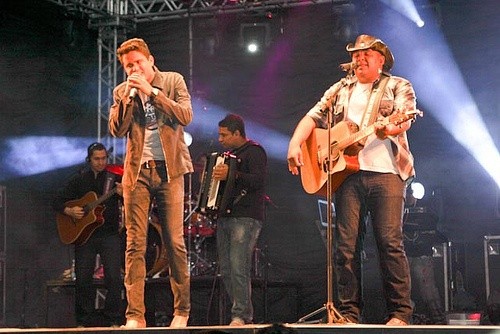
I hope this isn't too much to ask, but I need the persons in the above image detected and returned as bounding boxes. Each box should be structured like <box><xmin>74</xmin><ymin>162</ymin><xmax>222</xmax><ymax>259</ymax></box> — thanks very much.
<box><xmin>53</xmin><ymin>142</ymin><xmax>123</xmax><ymax>328</ymax></box>
<box><xmin>109</xmin><ymin>37</ymin><xmax>195</xmax><ymax>330</ymax></box>
<box><xmin>198</xmin><ymin>114</ymin><xmax>268</xmax><ymax>326</ymax></box>
<box><xmin>287</xmin><ymin>34</ymin><xmax>416</xmax><ymax>326</ymax></box>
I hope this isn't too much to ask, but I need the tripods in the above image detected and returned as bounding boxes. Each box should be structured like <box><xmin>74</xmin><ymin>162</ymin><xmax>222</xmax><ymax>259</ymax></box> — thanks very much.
<box><xmin>296</xmin><ymin>73</ymin><xmax>352</xmax><ymax>327</ymax></box>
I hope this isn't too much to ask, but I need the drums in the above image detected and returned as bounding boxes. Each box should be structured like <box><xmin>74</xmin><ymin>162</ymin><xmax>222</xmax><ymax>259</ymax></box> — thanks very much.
<box><xmin>119</xmin><ymin>222</ymin><xmax>167</xmax><ymax>278</ymax></box>
<box><xmin>183</xmin><ymin>191</ymin><xmax>216</xmax><ymax>237</ymax></box>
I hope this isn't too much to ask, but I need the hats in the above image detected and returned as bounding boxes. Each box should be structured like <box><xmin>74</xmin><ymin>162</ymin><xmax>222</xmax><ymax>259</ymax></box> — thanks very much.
<box><xmin>346</xmin><ymin>34</ymin><xmax>394</xmax><ymax>71</ymax></box>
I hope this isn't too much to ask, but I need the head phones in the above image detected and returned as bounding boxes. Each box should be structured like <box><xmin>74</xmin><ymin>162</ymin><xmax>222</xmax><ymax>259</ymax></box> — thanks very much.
<box><xmin>86</xmin><ymin>143</ymin><xmax>101</xmax><ymax>162</ymax></box>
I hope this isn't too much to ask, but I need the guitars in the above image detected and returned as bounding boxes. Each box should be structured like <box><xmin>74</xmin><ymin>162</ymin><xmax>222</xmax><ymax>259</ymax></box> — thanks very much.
<box><xmin>299</xmin><ymin>106</ymin><xmax>416</xmax><ymax>196</ymax></box>
<box><xmin>56</xmin><ymin>186</ymin><xmax>117</xmax><ymax>247</ymax></box>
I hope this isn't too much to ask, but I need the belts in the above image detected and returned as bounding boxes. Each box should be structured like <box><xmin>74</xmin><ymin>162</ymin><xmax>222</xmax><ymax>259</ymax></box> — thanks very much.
<box><xmin>141</xmin><ymin>160</ymin><xmax>166</xmax><ymax>169</ymax></box>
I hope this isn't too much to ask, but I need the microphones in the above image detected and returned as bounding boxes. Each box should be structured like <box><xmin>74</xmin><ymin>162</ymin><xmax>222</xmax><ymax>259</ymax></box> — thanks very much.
<box><xmin>129</xmin><ymin>87</ymin><xmax>137</xmax><ymax>99</ymax></box>
<box><xmin>339</xmin><ymin>61</ymin><xmax>357</xmax><ymax>71</ymax></box>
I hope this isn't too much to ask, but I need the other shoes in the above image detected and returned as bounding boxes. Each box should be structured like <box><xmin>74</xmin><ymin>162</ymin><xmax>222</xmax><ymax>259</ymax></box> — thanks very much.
<box><xmin>170</xmin><ymin>315</ymin><xmax>189</xmax><ymax>328</ymax></box>
<box><xmin>121</xmin><ymin>319</ymin><xmax>146</xmax><ymax>328</ymax></box>
<box><xmin>386</xmin><ymin>317</ymin><xmax>407</xmax><ymax>326</ymax></box>
<box><xmin>229</xmin><ymin>317</ymin><xmax>245</xmax><ymax>326</ymax></box>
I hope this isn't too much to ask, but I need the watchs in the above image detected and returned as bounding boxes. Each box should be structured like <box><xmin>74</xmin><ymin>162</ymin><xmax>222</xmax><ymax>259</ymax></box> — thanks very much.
<box><xmin>150</xmin><ymin>88</ymin><xmax>159</xmax><ymax>102</ymax></box>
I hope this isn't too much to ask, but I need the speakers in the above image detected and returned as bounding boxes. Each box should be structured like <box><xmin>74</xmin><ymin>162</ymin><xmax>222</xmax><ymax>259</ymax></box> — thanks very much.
<box><xmin>359</xmin><ymin>239</ymin><xmax>457</xmax><ymax>326</ymax></box>
